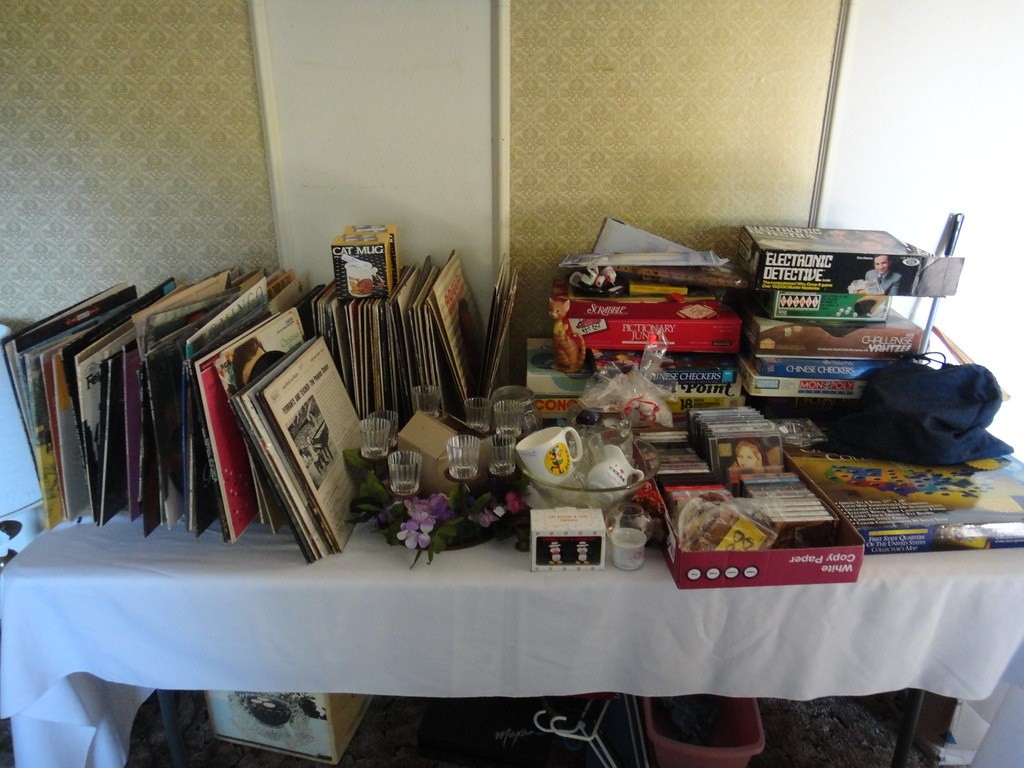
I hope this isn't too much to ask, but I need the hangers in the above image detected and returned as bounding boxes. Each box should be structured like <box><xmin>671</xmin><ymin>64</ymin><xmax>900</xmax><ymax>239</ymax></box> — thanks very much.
<box><xmin>533</xmin><ymin>692</ymin><xmax>641</xmax><ymax>768</ymax></box>
<box><xmin>550</xmin><ymin>695</ymin><xmax>650</xmax><ymax>768</ymax></box>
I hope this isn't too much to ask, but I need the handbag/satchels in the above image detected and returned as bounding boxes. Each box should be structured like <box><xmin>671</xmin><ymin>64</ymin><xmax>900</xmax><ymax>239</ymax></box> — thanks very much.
<box><xmin>806</xmin><ymin>346</ymin><xmax>1016</xmax><ymax>468</ymax></box>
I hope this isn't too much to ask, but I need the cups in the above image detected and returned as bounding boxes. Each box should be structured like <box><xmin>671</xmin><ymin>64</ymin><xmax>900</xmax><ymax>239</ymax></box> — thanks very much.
<box><xmin>464</xmin><ymin>397</ymin><xmax>492</xmax><ymax>433</ymax></box>
<box><xmin>368</xmin><ymin>410</ymin><xmax>398</xmax><ymax>447</ymax></box>
<box><xmin>515</xmin><ymin>426</ymin><xmax>583</xmax><ymax>485</ymax></box>
<box><xmin>494</xmin><ymin>400</ymin><xmax>523</xmax><ymax>438</ymax></box>
<box><xmin>411</xmin><ymin>386</ymin><xmax>439</xmax><ymax>418</ymax></box>
<box><xmin>613</xmin><ymin>528</ymin><xmax>647</xmax><ymax>571</ymax></box>
<box><xmin>489</xmin><ymin>436</ymin><xmax>516</xmax><ymax>475</ymax></box>
<box><xmin>358</xmin><ymin>419</ymin><xmax>391</xmax><ymax>458</ymax></box>
<box><xmin>388</xmin><ymin>451</ymin><xmax>422</xmax><ymax>495</ymax></box>
<box><xmin>447</xmin><ymin>436</ymin><xmax>480</xmax><ymax>479</ymax></box>
<box><xmin>588</xmin><ymin>445</ymin><xmax>644</xmax><ymax>505</ymax></box>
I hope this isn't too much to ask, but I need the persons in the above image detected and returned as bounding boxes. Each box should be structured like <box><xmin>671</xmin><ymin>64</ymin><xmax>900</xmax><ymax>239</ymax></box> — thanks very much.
<box><xmin>863</xmin><ymin>253</ymin><xmax>904</xmax><ymax>298</ymax></box>
<box><xmin>234</xmin><ymin>339</ymin><xmax>267</xmax><ymax>393</ymax></box>
<box><xmin>727</xmin><ymin>441</ymin><xmax>771</xmax><ymax>468</ymax></box>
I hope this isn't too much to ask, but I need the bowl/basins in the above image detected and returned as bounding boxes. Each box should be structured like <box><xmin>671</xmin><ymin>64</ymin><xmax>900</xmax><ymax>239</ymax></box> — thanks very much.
<box><xmin>512</xmin><ymin>434</ymin><xmax>660</xmax><ymax>514</ymax></box>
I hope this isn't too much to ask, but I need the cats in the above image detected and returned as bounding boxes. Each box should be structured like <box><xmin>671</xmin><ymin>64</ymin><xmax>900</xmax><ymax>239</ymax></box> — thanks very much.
<box><xmin>546</xmin><ymin>298</ymin><xmax>587</xmax><ymax>373</ymax></box>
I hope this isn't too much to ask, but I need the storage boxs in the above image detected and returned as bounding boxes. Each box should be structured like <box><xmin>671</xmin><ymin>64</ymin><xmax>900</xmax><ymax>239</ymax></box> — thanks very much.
<box><xmin>530</xmin><ymin>506</ymin><xmax>606</xmax><ymax>572</ymax></box>
<box><xmin>632</xmin><ymin>427</ymin><xmax>865</xmax><ymax>590</ymax></box>
<box><xmin>642</xmin><ymin>696</ymin><xmax>766</xmax><ymax>768</ymax></box>
<box><xmin>526</xmin><ymin>216</ymin><xmax>965</xmax><ymax>419</ymax></box>
<box><xmin>396</xmin><ymin>409</ymin><xmax>489</xmax><ymax>499</ymax></box>
<box><xmin>330</xmin><ymin>223</ymin><xmax>402</xmax><ymax>299</ymax></box>
<box><xmin>783</xmin><ymin>444</ymin><xmax>1024</xmax><ymax>555</ymax></box>
<box><xmin>203</xmin><ymin>690</ymin><xmax>375</xmax><ymax>765</ymax></box>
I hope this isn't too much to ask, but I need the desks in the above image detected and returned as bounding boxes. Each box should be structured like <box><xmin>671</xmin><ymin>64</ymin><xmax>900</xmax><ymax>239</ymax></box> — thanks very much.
<box><xmin>0</xmin><ymin>505</ymin><xmax>1024</xmax><ymax>768</ymax></box>
